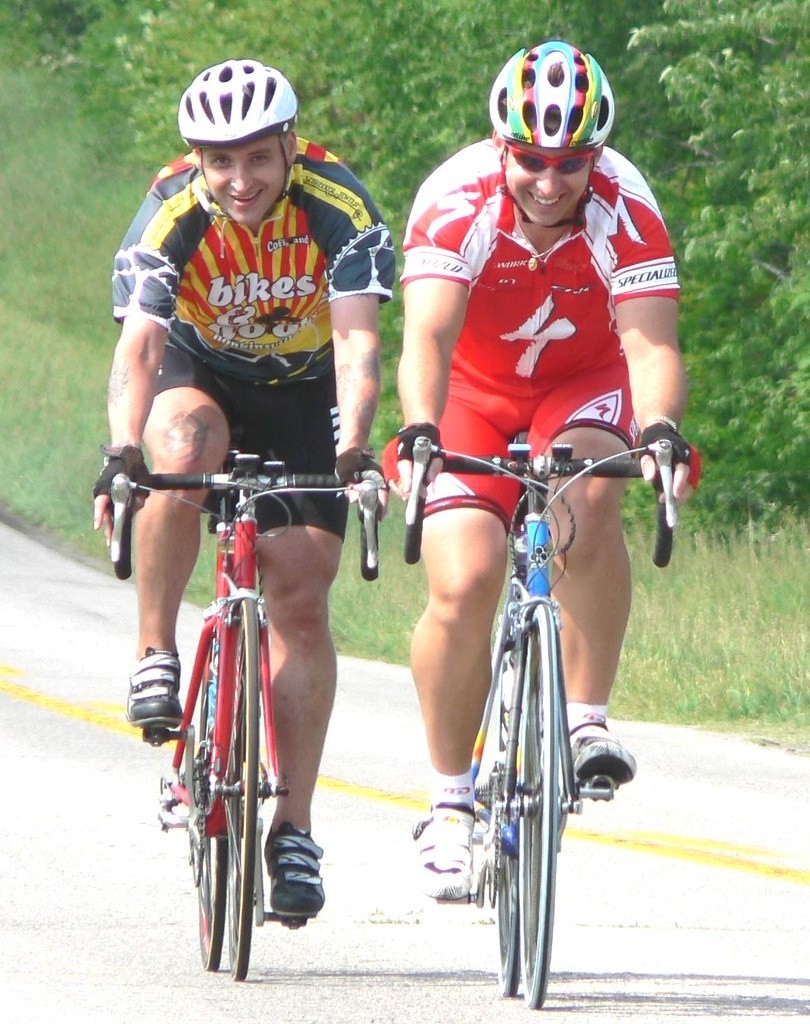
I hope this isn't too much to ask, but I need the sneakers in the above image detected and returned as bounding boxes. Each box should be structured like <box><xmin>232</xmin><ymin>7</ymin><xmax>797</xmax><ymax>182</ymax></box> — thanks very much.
<box><xmin>567</xmin><ymin>702</ymin><xmax>637</xmax><ymax>783</ymax></box>
<box><xmin>126</xmin><ymin>647</ymin><xmax>183</xmax><ymax>728</ymax></box>
<box><xmin>263</xmin><ymin>822</ymin><xmax>326</xmax><ymax>918</ymax></box>
<box><xmin>411</xmin><ymin>798</ymin><xmax>476</xmax><ymax>901</ymax></box>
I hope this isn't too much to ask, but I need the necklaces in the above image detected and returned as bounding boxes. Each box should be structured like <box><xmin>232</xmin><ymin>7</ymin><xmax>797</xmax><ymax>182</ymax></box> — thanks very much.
<box><xmin>514</xmin><ymin>213</ymin><xmax>571</xmax><ymax>272</ymax></box>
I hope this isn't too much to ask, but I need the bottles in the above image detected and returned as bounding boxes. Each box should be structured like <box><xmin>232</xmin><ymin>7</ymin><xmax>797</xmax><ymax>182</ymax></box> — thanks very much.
<box><xmin>500</xmin><ymin>641</ymin><xmax>514</xmax><ymax>752</ymax></box>
<box><xmin>208</xmin><ymin>644</ymin><xmax>220</xmax><ymax>733</ymax></box>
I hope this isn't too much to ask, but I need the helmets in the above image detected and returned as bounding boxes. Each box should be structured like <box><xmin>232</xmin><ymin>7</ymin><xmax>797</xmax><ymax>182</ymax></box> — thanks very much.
<box><xmin>177</xmin><ymin>60</ymin><xmax>299</xmax><ymax>149</ymax></box>
<box><xmin>488</xmin><ymin>39</ymin><xmax>616</xmax><ymax>149</ymax></box>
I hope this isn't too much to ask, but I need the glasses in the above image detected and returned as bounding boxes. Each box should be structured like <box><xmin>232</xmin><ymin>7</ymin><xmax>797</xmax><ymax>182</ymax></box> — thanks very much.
<box><xmin>499</xmin><ymin>141</ymin><xmax>597</xmax><ymax>175</ymax></box>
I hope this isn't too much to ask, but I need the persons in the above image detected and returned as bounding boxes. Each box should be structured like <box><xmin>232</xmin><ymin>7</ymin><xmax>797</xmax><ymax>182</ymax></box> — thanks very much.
<box><xmin>376</xmin><ymin>40</ymin><xmax>701</xmax><ymax>902</ymax></box>
<box><xmin>93</xmin><ymin>56</ymin><xmax>396</xmax><ymax>919</ymax></box>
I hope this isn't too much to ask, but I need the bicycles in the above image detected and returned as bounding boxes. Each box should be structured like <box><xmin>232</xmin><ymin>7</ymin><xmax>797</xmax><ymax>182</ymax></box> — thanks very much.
<box><xmin>402</xmin><ymin>431</ymin><xmax>682</xmax><ymax>1012</ymax></box>
<box><xmin>104</xmin><ymin>448</ymin><xmax>391</xmax><ymax>983</ymax></box>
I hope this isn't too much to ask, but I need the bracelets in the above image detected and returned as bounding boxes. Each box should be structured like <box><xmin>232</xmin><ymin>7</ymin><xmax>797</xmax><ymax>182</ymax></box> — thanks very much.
<box><xmin>646</xmin><ymin>415</ymin><xmax>677</xmax><ymax>431</ymax></box>
